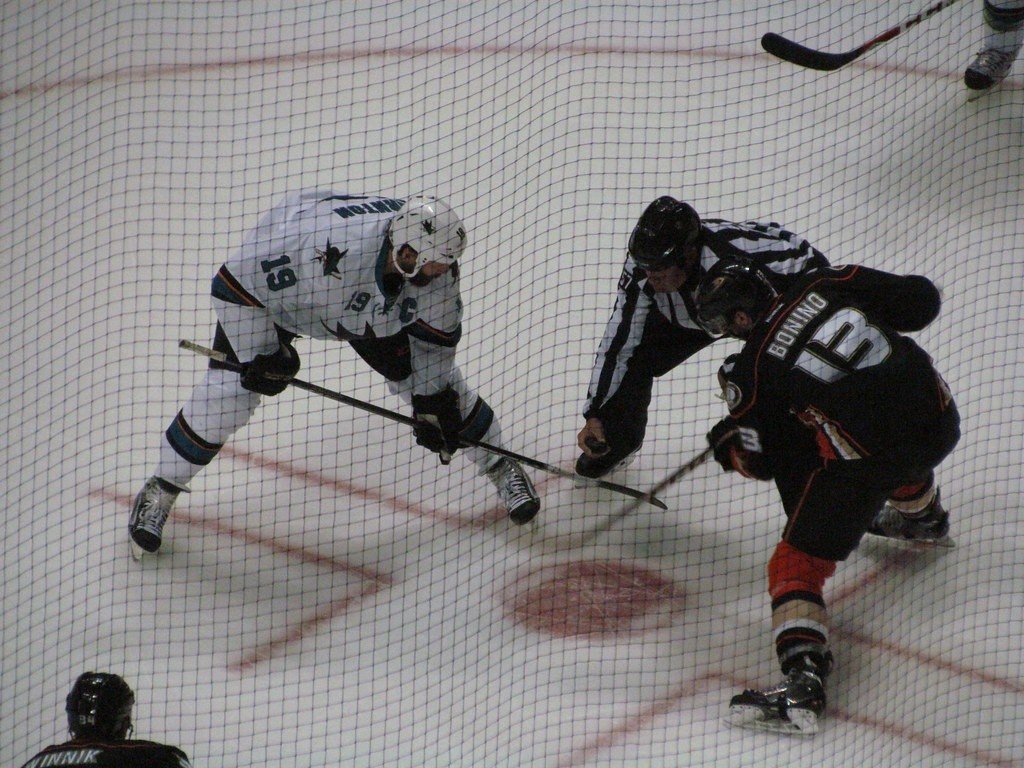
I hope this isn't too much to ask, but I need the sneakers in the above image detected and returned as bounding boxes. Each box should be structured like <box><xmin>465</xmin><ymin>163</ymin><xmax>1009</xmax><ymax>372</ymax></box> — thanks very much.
<box><xmin>576</xmin><ymin>436</ymin><xmax>644</xmax><ymax>490</ymax></box>
<box><xmin>868</xmin><ymin>487</ymin><xmax>958</xmax><ymax>548</ymax></box>
<box><xmin>127</xmin><ymin>474</ymin><xmax>193</xmax><ymax>560</ymax></box>
<box><xmin>963</xmin><ymin>47</ymin><xmax>1018</xmax><ymax>102</ymax></box>
<box><xmin>477</xmin><ymin>450</ymin><xmax>541</xmax><ymax>535</ymax></box>
<box><xmin>724</xmin><ymin>651</ymin><xmax>834</xmax><ymax>735</ymax></box>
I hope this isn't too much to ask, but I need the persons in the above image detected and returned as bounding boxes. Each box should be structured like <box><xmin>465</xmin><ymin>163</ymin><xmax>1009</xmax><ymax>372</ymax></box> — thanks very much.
<box><xmin>575</xmin><ymin>195</ymin><xmax>829</xmax><ymax>478</ymax></box>
<box><xmin>692</xmin><ymin>257</ymin><xmax>961</xmax><ymax>735</ymax></box>
<box><xmin>965</xmin><ymin>0</ymin><xmax>1024</xmax><ymax>101</ymax></box>
<box><xmin>129</xmin><ymin>191</ymin><xmax>539</xmax><ymax>553</ymax></box>
<box><xmin>20</xmin><ymin>671</ymin><xmax>195</xmax><ymax>768</ymax></box>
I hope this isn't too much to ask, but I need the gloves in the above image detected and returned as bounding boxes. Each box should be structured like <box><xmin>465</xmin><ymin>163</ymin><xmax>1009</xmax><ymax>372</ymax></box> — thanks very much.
<box><xmin>706</xmin><ymin>414</ymin><xmax>750</xmax><ymax>473</ymax></box>
<box><xmin>238</xmin><ymin>341</ymin><xmax>300</xmax><ymax>396</ymax></box>
<box><xmin>410</xmin><ymin>384</ymin><xmax>462</xmax><ymax>465</ymax></box>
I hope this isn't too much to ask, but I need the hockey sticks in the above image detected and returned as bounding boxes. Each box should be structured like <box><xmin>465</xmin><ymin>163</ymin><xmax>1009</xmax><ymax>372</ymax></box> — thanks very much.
<box><xmin>760</xmin><ymin>0</ymin><xmax>961</xmax><ymax>72</ymax></box>
<box><xmin>178</xmin><ymin>339</ymin><xmax>668</xmax><ymax>511</ymax></box>
<box><xmin>481</xmin><ymin>447</ymin><xmax>716</xmax><ymax>561</ymax></box>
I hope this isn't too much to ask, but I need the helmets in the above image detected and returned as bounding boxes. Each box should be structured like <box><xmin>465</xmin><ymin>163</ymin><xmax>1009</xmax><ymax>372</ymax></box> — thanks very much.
<box><xmin>628</xmin><ymin>196</ymin><xmax>703</xmax><ymax>277</ymax></box>
<box><xmin>64</xmin><ymin>670</ymin><xmax>134</xmax><ymax>743</ymax></box>
<box><xmin>390</xmin><ymin>195</ymin><xmax>468</xmax><ymax>279</ymax></box>
<box><xmin>698</xmin><ymin>262</ymin><xmax>777</xmax><ymax>334</ymax></box>
